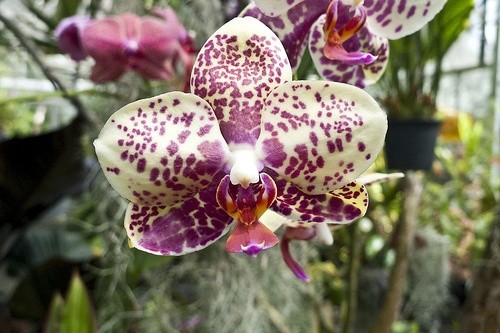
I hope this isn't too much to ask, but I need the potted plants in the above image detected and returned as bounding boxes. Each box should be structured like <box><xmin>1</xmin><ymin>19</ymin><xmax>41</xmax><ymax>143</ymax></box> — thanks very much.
<box><xmin>372</xmin><ymin>0</ymin><xmax>477</xmax><ymax>172</ymax></box>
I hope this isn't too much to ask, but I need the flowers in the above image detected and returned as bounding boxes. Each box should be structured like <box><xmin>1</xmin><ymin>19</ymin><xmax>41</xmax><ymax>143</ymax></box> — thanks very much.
<box><xmin>51</xmin><ymin>0</ymin><xmax>452</xmax><ymax>283</ymax></box>
